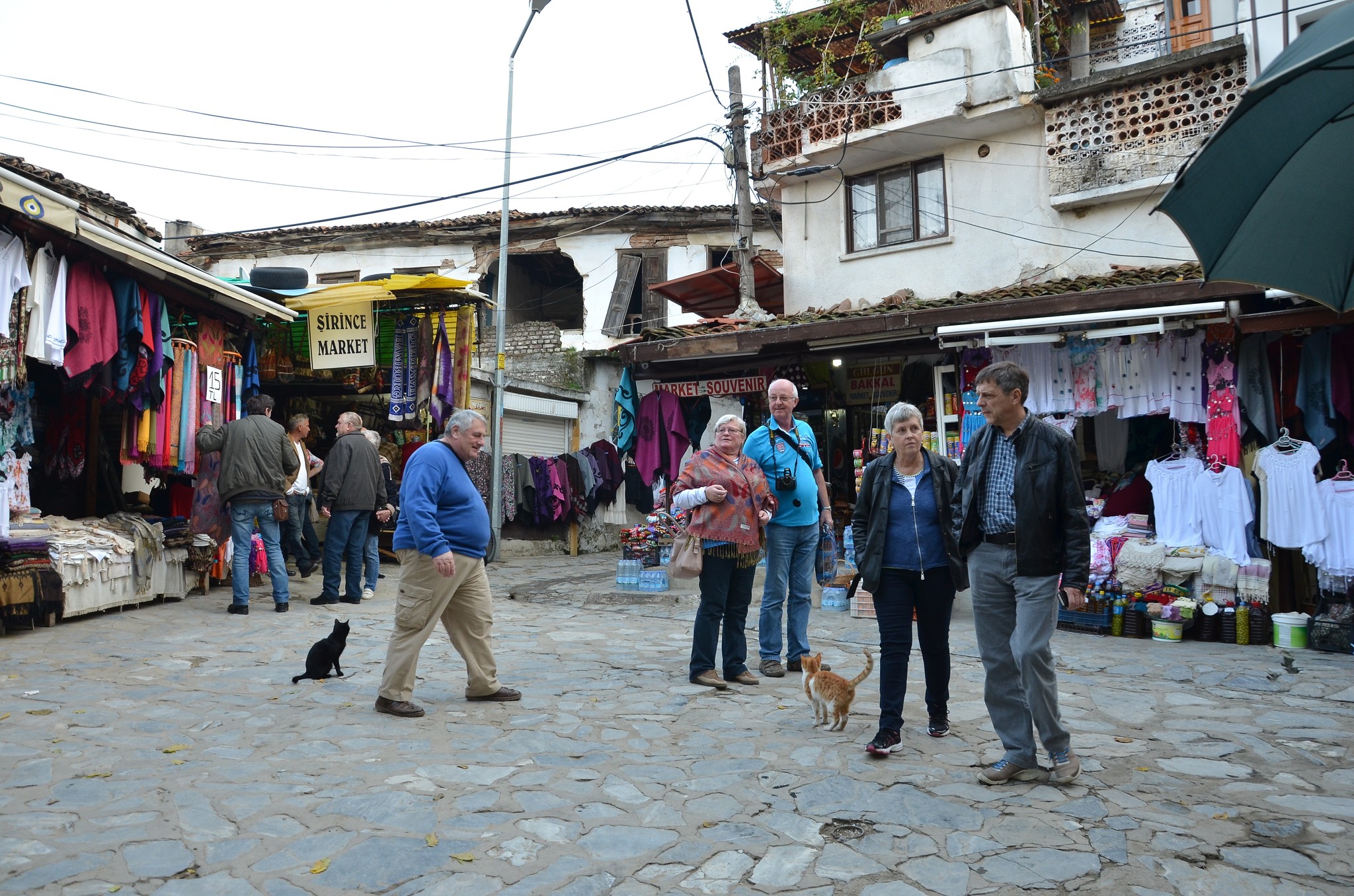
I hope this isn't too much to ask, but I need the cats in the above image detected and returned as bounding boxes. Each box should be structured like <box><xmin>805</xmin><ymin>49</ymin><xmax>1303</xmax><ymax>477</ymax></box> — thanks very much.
<box><xmin>800</xmin><ymin>649</ymin><xmax>873</xmax><ymax>731</ymax></box>
<box><xmin>292</xmin><ymin>619</ymin><xmax>350</xmax><ymax>683</ymax></box>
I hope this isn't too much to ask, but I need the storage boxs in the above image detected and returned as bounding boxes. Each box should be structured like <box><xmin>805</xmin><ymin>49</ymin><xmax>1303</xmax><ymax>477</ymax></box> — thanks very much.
<box><xmin>849</xmin><ymin>578</ymin><xmax>878</xmax><ymax>619</ymax></box>
<box><xmin>621</xmin><ymin>544</ymin><xmax>660</xmax><ymax>566</ymax></box>
<box><xmin>1056</xmin><ymin>595</ymin><xmax>1114</xmax><ymax>628</ymax></box>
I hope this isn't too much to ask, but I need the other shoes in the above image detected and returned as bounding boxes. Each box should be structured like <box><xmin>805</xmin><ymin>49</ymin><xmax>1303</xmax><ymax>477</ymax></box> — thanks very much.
<box><xmin>364</xmin><ymin>568</ymin><xmax>385</xmax><ymax>578</ymax></box>
<box><xmin>375</xmin><ymin>695</ymin><xmax>425</xmax><ymax>717</ymax></box>
<box><xmin>465</xmin><ymin>686</ymin><xmax>521</xmax><ymax>701</ymax></box>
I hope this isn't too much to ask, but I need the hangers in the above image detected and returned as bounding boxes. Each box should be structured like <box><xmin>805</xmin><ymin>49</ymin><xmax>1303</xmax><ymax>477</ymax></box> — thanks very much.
<box><xmin>1203</xmin><ymin>324</ymin><xmax>1235</xmax><ymax>418</ymax></box>
<box><xmin>373</xmin><ymin>295</ymin><xmax>448</xmax><ymax>322</ymax></box>
<box><xmin>481</xmin><ymin>435</ymin><xmax>615</xmax><ymax>471</ymax></box>
<box><xmin>0</xmin><ymin>218</ymin><xmax>58</xmax><ymax>261</ymax></box>
<box><xmin>1154</xmin><ymin>426</ymin><xmax>1354</xmax><ymax>494</ymax></box>
<box><xmin>965</xmin><ymin>328</ymin><xmax>1203</xmax><ymax>347</ymax></box>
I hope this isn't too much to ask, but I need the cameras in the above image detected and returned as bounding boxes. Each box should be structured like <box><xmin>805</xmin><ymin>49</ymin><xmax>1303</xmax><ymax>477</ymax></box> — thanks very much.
<box><xmin>775</xmin><ymin>468</ymin><xmax>797</xmax><ymax>491</ymax></box>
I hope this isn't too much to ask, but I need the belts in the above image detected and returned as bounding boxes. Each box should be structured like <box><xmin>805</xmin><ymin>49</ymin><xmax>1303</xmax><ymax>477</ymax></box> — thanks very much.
<box><xmin>982</xmin><ymin>529</ymin><xmax>1015</xmax><ymax>545</ymax></box>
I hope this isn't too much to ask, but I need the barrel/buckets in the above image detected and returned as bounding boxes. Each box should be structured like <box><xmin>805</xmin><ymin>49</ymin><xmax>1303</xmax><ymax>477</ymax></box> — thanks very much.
<box><xmin>1271</xmin><ymin>612</ymin><xmax>1310</xmax><ymax>649</ymax></box>
<box><xmin>1151</xmin><ymin>616</ymin><xmax>1184</xmax><ymax>643</ymax></box>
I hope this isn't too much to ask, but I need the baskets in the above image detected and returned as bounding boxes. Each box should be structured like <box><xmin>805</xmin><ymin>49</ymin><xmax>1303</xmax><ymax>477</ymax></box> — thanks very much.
<box><xmin>1307</xmin><ymin>595</ymin><xmax>1354</xmax><ymax>654</ymax></box>
<box><xmin>833</xmin><ymin>559</ymin><xmax>857</xmax><ymax>584</ymax></box>
<box><xmin>1055</xmin><ymin>620</ymin><xmax>1108</xmax><ymax>637</ymax></box>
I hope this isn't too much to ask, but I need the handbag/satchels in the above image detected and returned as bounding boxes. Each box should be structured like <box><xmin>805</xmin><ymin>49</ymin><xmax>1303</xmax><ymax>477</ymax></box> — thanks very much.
<box><xmin>274</xmin><ymin>499</ymin><xmax>289</xmax><ymax>521</ymax></box>
<box><xmin>658</xmin><ymin>508</ymin><xmax>702</xmax><ymax>579</ymax></box>
<box><xmin>308</xmin><ymin>497</ymin><xmax>319</xmax><ymax>524</ymax></box>
<box><xmin>814</xmin><ymin>523</ymin><xmax>837</xmax><ymax>588</ymax></box>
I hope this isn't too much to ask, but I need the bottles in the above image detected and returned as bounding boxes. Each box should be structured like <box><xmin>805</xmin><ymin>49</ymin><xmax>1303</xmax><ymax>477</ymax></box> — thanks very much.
<box><xmin>756</xmin><ymin>545</ymin><xmax>766</xmax><ymax>566</ymax></box>
<box><xmin>616</xmin><ymin>546</ymin><xmax>675</xmax><ymax>592</ymax></box>
<box><xmin>1196</xmin><ymin>592</ymin><xmax>1266</xmax><ymax>645</ymax></box>
<box><xmin>1070</xmin><ymin>578</ymin><xmax>1151</xmax><ymax>638</ymax></box>
<box><xmin>821</xmin><ymin>585</ymin><xmax>850</xmax><ymax>612</ymax></box>
<box><xmin>833</xmin><ymin>522</ymin><xmax>857</xmax><ymax>568</ymax></box>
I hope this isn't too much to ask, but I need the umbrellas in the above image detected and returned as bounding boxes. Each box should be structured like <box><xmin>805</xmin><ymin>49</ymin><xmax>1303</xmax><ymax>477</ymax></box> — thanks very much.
<box><xmin>1148</xmin><ymin>0</ymin><xmax>1353</xmax><ymax>320</ymax></box>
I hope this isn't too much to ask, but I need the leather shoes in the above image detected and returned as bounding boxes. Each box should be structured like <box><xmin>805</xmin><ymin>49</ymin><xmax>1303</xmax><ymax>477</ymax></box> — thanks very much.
<box><xmin>310</xmin><ymin>594</ymin><xmax>340</xmax><ymax>605</ymax></box>
<box><xmin>275</xmin><ymin>602</ymin><xmax>289</xmax><ymax>612</ymax></box>
<box><xmin>301</xmin><ymin>563</ymin><xmax>318</xmax><ymax>578</ymax></box>
<box><xmin>340</xmin><ymin>593</ymin><xmax>361</xmax><ymax>604</ymax></box>
<box><xmin>285</xmin><ymin>568</ymin><xmax>296</xmax><ymax>576</ymax></box>
<box><xmin>310</xmin><ymin>557</ymin><xmax>323</xmax><ymax>564</ymax></box>
<box><xmin>227</xmin><ymin>604</ymin><xmax>248</xmax><ymax>615</ymax></box>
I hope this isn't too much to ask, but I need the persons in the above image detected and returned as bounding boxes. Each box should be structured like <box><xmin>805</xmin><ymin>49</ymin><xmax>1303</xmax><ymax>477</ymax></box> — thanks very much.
<box><xmin>299</xmin><ymin>448</ymin><xmax>325</xmax><ymax>564</ymax></box>
<box><xmin>195</xmin><ymin>392</ymin><xmax>300</xmax><ymax>615</ymax></box>
<box><xmin>668</xmin><ymin>414</ymin><xmax>780</xmax><ymax>688</ymax></box>
<box><xmin>740</xmin><ymin>378</ymin><xmax>834</xmax><ymax>678</ymax></box>
<box><xmin>309</xmin><ymin>411</ymin><xmax>391</xmax><ymax>606</ymax></box>
<box><xmin>277</xmin><ymin>412</ymin><xmax>319</xmax><ymax>579</ymax></box>
<box><xmin>845</xmin><ymin>401</ymin><xmax>970</xmax><ymax>755</ymax></box>
<box><xmin>948</xmin><ymin>360</ymin><xmax>1092</xmax><ymax>786</ymax></box>
<box><xmin>374</xmin><ymin>408</ymin><xmax>523</xmax><ymax>718</ymax></box>
<box><xmin>338</xmin><ymin>430</ymin><xmax>398</xmax><ymax>600</ymax></box>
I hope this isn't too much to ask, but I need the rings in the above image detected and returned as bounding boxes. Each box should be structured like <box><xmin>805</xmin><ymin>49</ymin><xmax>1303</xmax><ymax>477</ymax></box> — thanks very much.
<box><xmin>322</xmin><ymin>510</ymin><xmax>325</xmax><ymax>513</ymax></box>
<box><xmin>763</xmin><ymin>521</ymin><xmax>765</xmax><ymax>524</ymax></box>
<box><xmin>718</xmin><ymin>495</ymin><xmax>720</xmax><ymax>498</ymax></box>
<box><xmin>718</xmin><ymin>498</ymin><xmax>720</xmax><ymax>501</ymax></box>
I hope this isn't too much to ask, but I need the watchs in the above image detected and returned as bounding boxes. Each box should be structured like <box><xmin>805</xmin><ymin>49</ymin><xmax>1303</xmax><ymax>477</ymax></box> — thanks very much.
<box><xmin>822</xmin><ymin>505</ymin><xmax>832</xmax><ymax>511</ymax></box>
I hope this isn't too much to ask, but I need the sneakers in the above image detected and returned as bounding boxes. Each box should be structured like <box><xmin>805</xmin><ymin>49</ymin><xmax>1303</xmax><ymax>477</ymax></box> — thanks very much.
<box><xmin>927</xmin><ymin>710</ymin><xmax>951</xmax><ymax>737</ymax></box>
<box><xmin>864</xmin><ymin>727</ymin><xmax>903</xmax><ymax>755</ymax></box>
<box><xmin>759</xmin><ymin>659</ymin><xmax>785</xmax><ymax>677</ymax></box>
<box><xmin>689</xmin><ymin>669</ymin><xmax>727</xmax><ymax>687</ymax></box>
<box><xmin>1048</xmin><ymin>743</ymin><xmax>1082</xmax><ymax>783</ymax></box>
<box><xmin>787</xmin><ymin>655</ymin><xmax>831</xmax><ymax>672</ymax></box>
<box><xmin>977</xmin><ymin>756</ymin><xmax>1039</xmax><ymax>784</ymax></box>
<box><xmin>728</xmin><ymin>670</ymin><xmax>759</xmax><ymax>685</ymax></box>
<box><xmin>361</xmin><ymin>588</ymin><xmax>374</xmax><ymax>599</ymax></box>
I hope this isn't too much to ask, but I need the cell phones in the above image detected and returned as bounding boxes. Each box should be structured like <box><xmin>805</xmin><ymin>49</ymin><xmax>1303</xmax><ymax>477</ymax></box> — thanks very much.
<box><xmin>1057</xmin><ymin>590</ymin><xmax>1069</xmax><ymax>607</ymax></box>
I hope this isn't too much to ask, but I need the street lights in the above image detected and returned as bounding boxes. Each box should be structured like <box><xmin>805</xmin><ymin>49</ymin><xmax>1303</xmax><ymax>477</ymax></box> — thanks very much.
<box><xmin>483</xmin><ymin>1</ymin><xmax>557</xmax><ymax>557</ymax></box>
